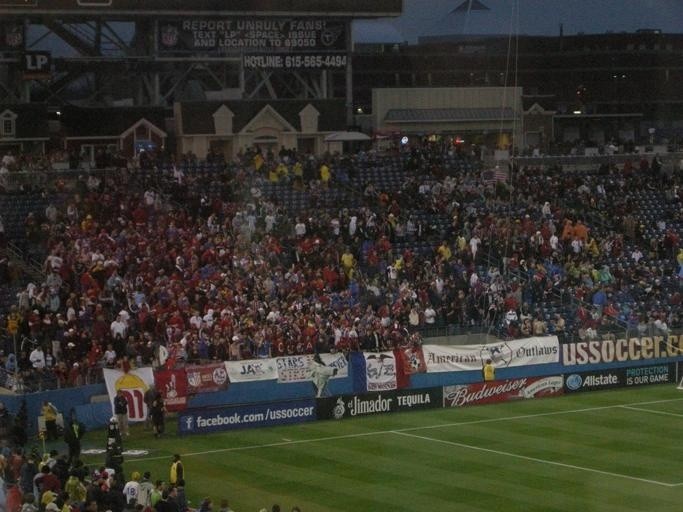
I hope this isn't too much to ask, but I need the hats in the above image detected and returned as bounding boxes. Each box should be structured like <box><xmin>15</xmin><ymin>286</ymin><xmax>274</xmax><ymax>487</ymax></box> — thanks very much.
<box><xmin>68</xmin><ymin>343</ymin><xmax>76</xmax><ymax>347</ymax></box>
<box><xmin>131</xmin><ymin>472</ymin><xmax>141</xmax><ymax>481</ymax></box>
<box><xmin>34</xmin><ymin>309</ymin><xmax>40</xmax><ymax>314</ymax></box>
<box><xmin>44</xmin><ymin>453</ymin><xmax>51</xmax><ymax>459</ymax></box>
<box><xmin>56</xmin><ymin>313</ymin><xmax>61</xmax><ymax>316</ymax></box>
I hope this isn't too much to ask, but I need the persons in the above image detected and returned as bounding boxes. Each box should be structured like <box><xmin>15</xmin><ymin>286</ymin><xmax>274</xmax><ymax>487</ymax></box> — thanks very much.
<box><xmin>115</xmin><ymin>389</ymin><xmax>129</xmax><ymax>436</ymax></box>
<box><xmin>258</xmin><ymin>504</ymin><xmax>280</xmax><ymax>512</ymax></box>
<box><xmin>292</xmin><ymin>506</ymin><xmax>301</xmax><ymax>512</ymax></box>
<box><xmin>1</xmin><ymin>399</ymin><xmax>190</xmax><ymax>511</ymax></box>
<box><xmin>199</xmin><ymin>496</ymin><xmax>232</xmax><ymax>511</ymax></box>
<box><xmin>144</xmin><ymin>383</ymin><xmax>168</xmax><ymax>438</ymax></box>
<box><xmin>1</xmin><ymin>138</ymin><xmax>683</xmax><ymax>382</ymax></box>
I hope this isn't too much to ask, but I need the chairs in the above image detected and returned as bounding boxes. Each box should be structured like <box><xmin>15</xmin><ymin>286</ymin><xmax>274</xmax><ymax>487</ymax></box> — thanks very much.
<box><xmin>0</xmin><ymin>144</ymin><xmax>683</xmax><ymax>391</ymax></box>
